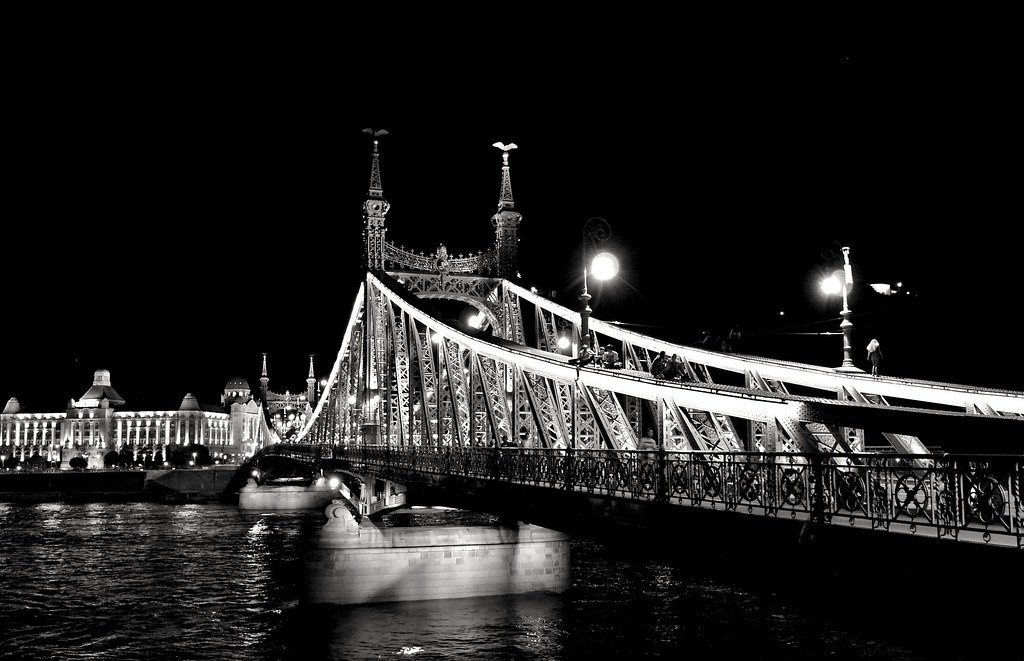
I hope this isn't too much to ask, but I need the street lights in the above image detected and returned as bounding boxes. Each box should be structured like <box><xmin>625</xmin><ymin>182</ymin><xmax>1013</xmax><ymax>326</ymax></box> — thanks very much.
<box><xmin>825</xmin><ymin>244</ymin><xmax>866</xmax><ymax>372</ymax></box>
<box><xmin>577</xmin><ymin>215</ymin><xmax>620</xmax><ymax>365</ymax></box>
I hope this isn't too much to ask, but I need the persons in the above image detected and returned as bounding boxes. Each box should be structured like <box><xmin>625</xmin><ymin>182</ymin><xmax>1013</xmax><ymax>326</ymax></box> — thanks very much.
<box><xmin>637</xmin><ymin>428</ymin><xmax>659</xmax><ymax>499</ymax></box>
<box><xmin>867</xmin><ymin>339</ymin><xmax>883</xmax><ymax>377</ymax></box>
<box><xmin>487</xmin><ymin>436</ymin><xmax>514</xmax><ymax>479</ymax></box>
<box><xmin>579</xmin><ymin>344</ymin><xmax>622</xmax><ymax>370</ymax></box>
<box><xmin>663</xmin><ymin>354</ymin><xmax>691</xmax><ymax>382</ymax></box>
<box><xmin>650</xmin><ymin>351</ymin><xmax>669</xmax><ymax>378</ymax></box>
